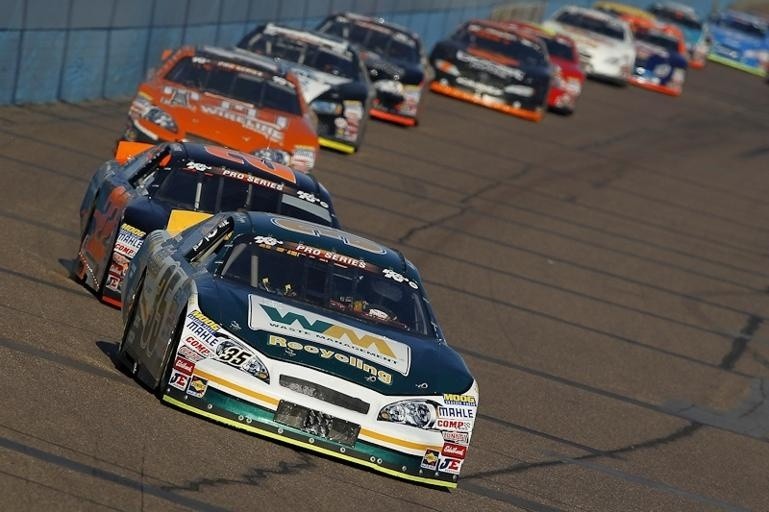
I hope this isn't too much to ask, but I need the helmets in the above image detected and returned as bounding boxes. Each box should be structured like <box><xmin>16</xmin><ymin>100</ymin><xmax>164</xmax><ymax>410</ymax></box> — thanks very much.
<box><xmin>371</xmin><ymin>277</ymin><xmax>403</xmax><ymax>303</ymax></box>
